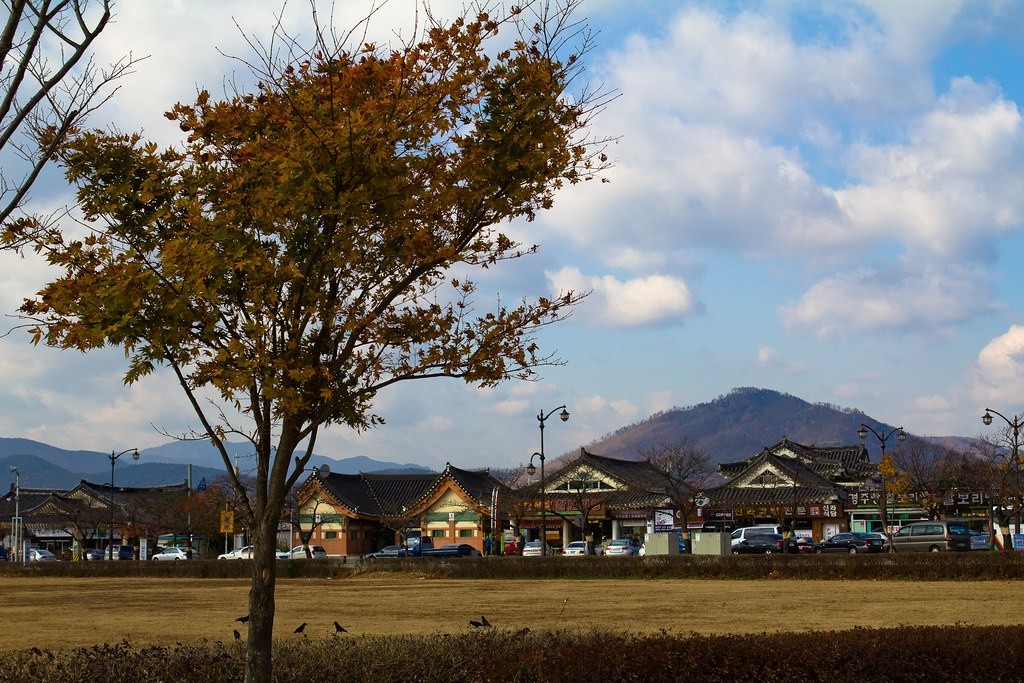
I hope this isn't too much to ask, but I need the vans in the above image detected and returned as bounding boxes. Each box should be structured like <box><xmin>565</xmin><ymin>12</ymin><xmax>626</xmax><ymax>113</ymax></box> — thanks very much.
<box><xmin>731</xmin><ymin>524</ymin><xmax>782</xmax><ymax>546</ymax></box>
<box><xmin>105</xmin><ymin>544</ymin><xmax>134</xmax><ymax>560</ymax></box>
<box><xmin>881</xmin><ymin>520</ymin><xmax>970</xmax><ymax>554</ymax></box>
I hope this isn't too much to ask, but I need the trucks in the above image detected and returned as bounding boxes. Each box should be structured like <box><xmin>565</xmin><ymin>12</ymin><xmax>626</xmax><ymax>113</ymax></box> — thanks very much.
<box><xmin>398</xmin><ymin>536</ymin><xmax>472</xmax><ymax>558</ymax></box>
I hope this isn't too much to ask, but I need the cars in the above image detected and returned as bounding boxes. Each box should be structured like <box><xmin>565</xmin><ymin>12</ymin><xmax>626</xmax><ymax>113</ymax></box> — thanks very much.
<box><xmin>522</xmin><ymin>539</ymin><xmax>552</xmax><ymax>556</ymax></box>
<box><xmin>31</xmin><ymin>549</ymin><xmax>57</xmax><ymax>562</ymax></box>
<box><xmin>287</xmin><ymin>544</ymin><xmax>326</xmax><ymax>560</ymax></box>
<box><xmin>606</xmin><ymin>540</ymin><xmax>639</xmax><ymax>555</ymax></box>
<box><xmin>274</xmin><ymin>548</ymin><xmax>290</xmax><ymax>559</ymax></box>
<box><xmin>814</xmin><ymin>533</ymin><xmax>886</xmax><ymax>555</ymax></box>
<box><xmin>949</xmin><ymin>528</ymin><xmax>991</xmax><ymax>550</ymax></box>
<box><xmin>732</xmin><ymin>534</ymin><xmax>784</xmax><ymax>555</ymax></box>
<box><xmin>152</xmin><ymin>546</ymin><xmax>200</xmax><ymax>560</ymax></box>
<box><xmin>562</xmin><ymin>540</ymin><xmax>590</xmax><ymax>556</ymax></box>
<box><xmin>365</xmin><ymin>546</ymin><xmax>400</xmax><ymax>559</ymax></box>
<box><xmin>441</xmin><ymin>544</ymin><xmax>483</xmax><ymax>558</ymax></box>
<box><xmin>788</xmin><ymin>537</ymin><xmax>815</xmax><ymax>553</ymax></box>
<box><xmin>80</xmin><ymin>549</ymin><xmax>104</xmax><ymax>561</ymax></box>
<box><xmin>216</xmin><ymin>545</ymin><xmax>255</xmax><ymax>561</ymax></box>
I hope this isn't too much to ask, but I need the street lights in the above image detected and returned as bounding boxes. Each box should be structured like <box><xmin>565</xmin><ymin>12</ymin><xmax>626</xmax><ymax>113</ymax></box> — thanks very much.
<box><xmin>526</xmin><ymin>404</ymin><xmax>571</xmax><ymax>555</ymax></box>
<box><xmin>982</xmin><ymin>406</ymin><xmax>1024</xmax><ymax>534</ymax></box>
<box><xmin>856</xmin><ymin>423</ymin><xmax>908</xmax><ymax>530</ymax></box>
<box><xmin>98</xmin><ymin>447</ymin><xmax>141</xmax><ymax>560</ymax></box>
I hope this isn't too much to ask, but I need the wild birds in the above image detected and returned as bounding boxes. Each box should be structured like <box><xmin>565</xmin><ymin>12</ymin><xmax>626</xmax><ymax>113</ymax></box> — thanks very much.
<box><xmin>333</xmin><ymin>622</ymin><xmax>347</xmax><ymax>634</ymax></box>
<box><xmin>233</xmin><ymin>629</ymin><xmax>240</xmax><ymax>640</ymax></box>
<box><xmin>235</xmin><ymin>615</ymin><xmax>249</xmax><ymax>623</ymax></box>
<box><xmin>470</xmin><ymin>615</ymin><xmax>491</xmax><ymax>628</ymax></box>
<box><xmin>293</xmin><ymin>623</ymin><xmax>306</xmax><ymax>634</ymax></box>
